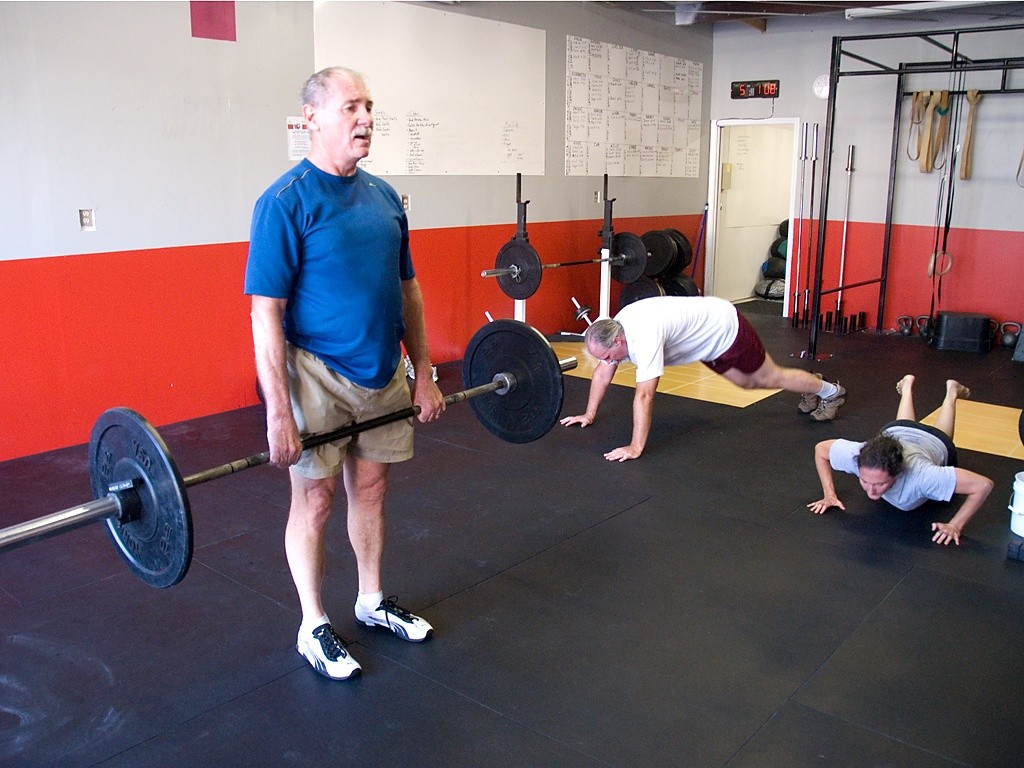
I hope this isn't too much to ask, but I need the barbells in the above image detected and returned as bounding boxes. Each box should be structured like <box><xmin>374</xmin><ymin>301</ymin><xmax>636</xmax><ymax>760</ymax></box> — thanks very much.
<box><xmin>480</xmin><ymin>231</ymin><xmax>649</xmax><ymax>301</ymax></box>
<box><xmin>0</xmin><ymin>318</ymin><xmax>580</xmax><ymax>587</ymax></box>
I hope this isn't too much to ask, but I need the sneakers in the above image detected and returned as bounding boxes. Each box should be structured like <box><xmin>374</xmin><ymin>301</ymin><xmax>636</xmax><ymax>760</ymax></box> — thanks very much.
<box><xmin>296</xmin><ymin>616</ymin><xmax>362</xmax><ymax>680</ymax></box>
<box><xmin>812</xmin><ymin>379</ymin><xmax>848</xmax><ymax>422</ymax></box>
<box><xmin>801</xmin><ymin>372</ymin><xmax>826</xmax><ymax>416</ymax></box>
<box><xmin>354</xmin><ymin>594</ymin><xmax>433</xmax><ymax>643</ymax></box>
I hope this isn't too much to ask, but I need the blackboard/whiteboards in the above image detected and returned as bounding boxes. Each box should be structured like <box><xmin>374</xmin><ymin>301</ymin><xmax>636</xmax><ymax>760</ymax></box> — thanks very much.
<box><xmin>313</xmin><ymin>1</ymin><xmax>546</xmax><ymax>176</ymax></box>
<box><xmin>565</xmin><ymin>35</ymin><xmax>703</xmax><ymax>178</ymax></box>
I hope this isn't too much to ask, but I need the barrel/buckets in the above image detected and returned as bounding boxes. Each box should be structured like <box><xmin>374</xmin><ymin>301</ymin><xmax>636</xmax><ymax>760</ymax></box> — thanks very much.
<box><xmin>1008</xmin><ymin>472</ymin><xmax>1024</xmax><ymax>538</ymax></box>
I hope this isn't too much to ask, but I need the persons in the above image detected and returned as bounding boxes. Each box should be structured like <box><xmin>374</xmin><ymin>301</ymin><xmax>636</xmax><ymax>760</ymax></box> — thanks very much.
<box><xmin>806</xmin><ymin>371</ymin><xmax>997</xmax><ymax>546</ymax></box>
<box><xmin>560</xmin><ymin>293</ymin><xmax>849</xmax><ymax>465</ymax></box>
<box><xmin>243</xmin><ymin>66</ymin><xmax>446</xmax><ymax>683</ymax></box>
